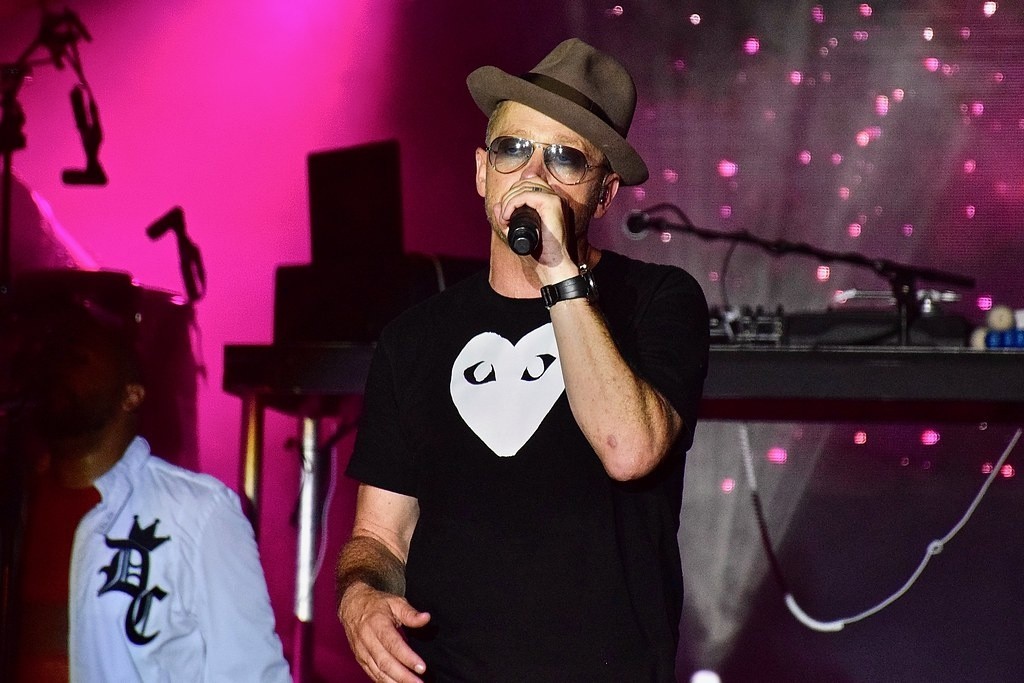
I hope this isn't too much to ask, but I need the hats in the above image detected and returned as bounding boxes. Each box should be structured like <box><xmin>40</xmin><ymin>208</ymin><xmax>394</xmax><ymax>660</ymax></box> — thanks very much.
<box><xmin>465</xmin><ymin>38</ymin><xmax>649</xmax><ymax>185</ymax></box>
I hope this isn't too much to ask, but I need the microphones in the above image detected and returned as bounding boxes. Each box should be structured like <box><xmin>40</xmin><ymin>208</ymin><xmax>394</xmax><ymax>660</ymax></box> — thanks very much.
<box><xmin>507</xmin><ymin>203</ymin><xmax>541</xmax><ymax>256</ymax></box>
<box><xmin>625</xmin><ymin>212</ymin><xmax>670</xmax><ymax>235</ymax></box>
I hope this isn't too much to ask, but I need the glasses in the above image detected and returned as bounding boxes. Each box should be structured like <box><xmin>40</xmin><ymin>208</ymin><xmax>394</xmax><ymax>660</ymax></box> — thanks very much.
<box><xmin>482</xmin><ymin>136</ymin><xmax>609</xmax><ymax>185</ymax></box>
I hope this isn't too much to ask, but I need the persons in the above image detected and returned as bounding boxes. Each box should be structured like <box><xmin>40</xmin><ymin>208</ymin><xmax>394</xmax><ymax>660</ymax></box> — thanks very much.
<box><xmin>0</xmin><ymin>285</ymin><xmax>297</xmax><ymax>683</ymax></box>
<box><xmin>335</xmin><ymin>36</ymin><xmax>710</xmax><ymax>683</ymax></box>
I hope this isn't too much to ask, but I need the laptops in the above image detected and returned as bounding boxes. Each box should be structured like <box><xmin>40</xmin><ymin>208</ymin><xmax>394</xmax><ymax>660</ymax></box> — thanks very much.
<box><xmin>304</xmin><ymin>136</ymin><xmax>458</xmax><ymax>266</ymax></box>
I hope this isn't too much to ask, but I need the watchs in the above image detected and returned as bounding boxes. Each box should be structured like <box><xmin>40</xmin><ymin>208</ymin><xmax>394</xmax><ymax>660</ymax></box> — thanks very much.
<box><xmin>540</xmin><ymin>261</ymin><xmax>600</xmax><ymax>308</ymax></box>
